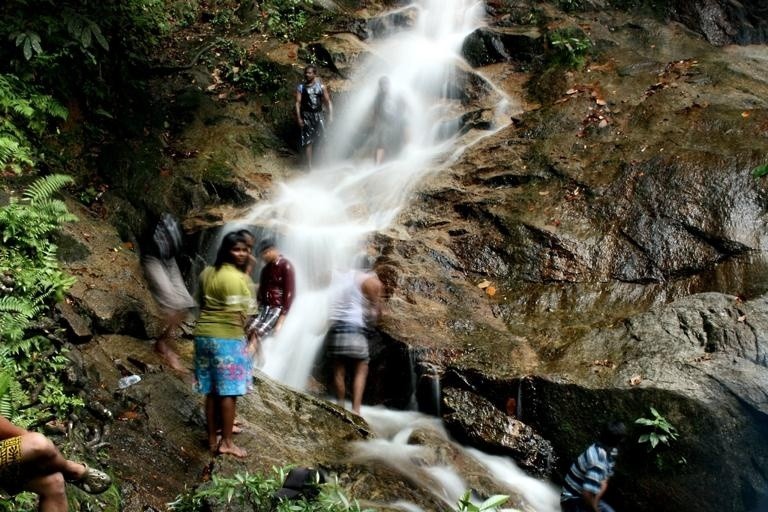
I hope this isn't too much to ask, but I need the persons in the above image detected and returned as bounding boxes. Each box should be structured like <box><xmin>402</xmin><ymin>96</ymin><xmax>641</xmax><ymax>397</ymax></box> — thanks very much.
<box><xmin>370</xmin><ymin>76</ymin><xmax>415</xmax><ymax>172</ymax></box>
<box><xmin>0</xmin><ymin>411</ymin><xmax>113</xmax><ymax>511</ymax></box>
<box><xmin>295</xmin><ymin>65</ymin><xmax>335</xmax><ymax>174</ymax></box>
<box><xmin>560</xmin><ymin>420</ymin><xmax>628</xmax><ymax>511</ymax></box>
<box><xmin>329</xmin><ymin>255</ymin><xmax>383</xmax><ymax>415</ymax></box>
<box><xmin>193</xmin><ymin>230</ymin><xmax>296</xmax><ymax>459</ymax></box>
<box><xmin>140</xmin><ymin>208</ymin><xmax>201</xmax><ymax>373</ymax></box>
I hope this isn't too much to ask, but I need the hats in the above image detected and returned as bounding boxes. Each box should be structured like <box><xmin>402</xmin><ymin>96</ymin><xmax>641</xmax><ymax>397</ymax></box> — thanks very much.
<box><xmin>274</xmin><ymin>470</ymin><xmax>325</xmax><ymax>501</ymax></box>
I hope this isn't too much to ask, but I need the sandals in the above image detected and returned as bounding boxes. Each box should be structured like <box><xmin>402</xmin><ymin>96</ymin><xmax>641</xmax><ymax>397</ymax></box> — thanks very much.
<box><xmin>66</xmin><ymin>463</ymin><xmax>112</xmax><ymax>495</ymax></box>
